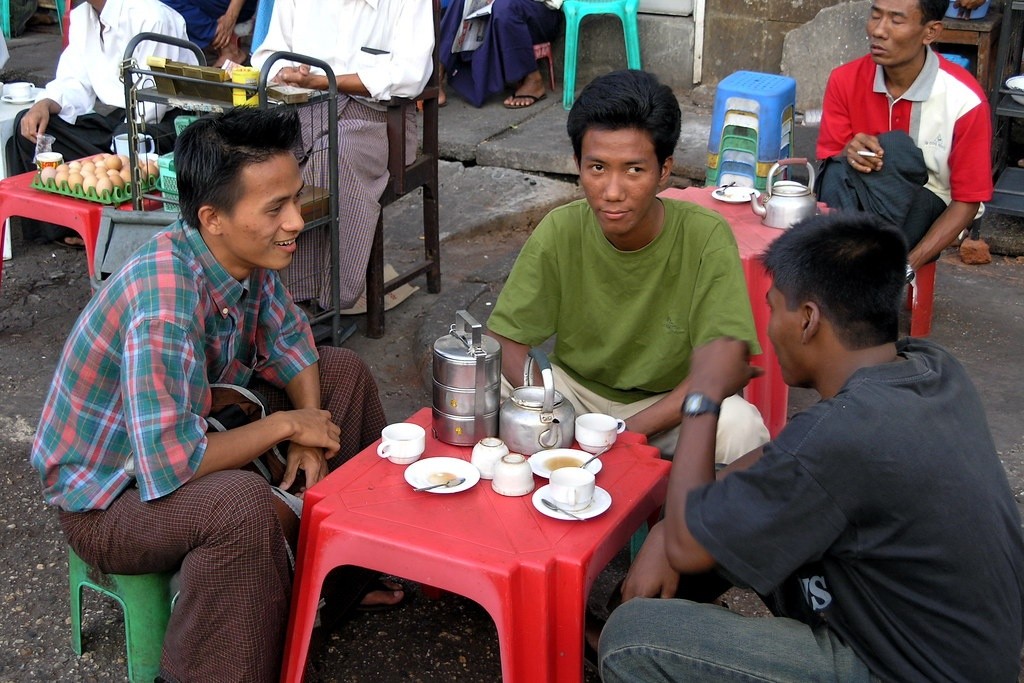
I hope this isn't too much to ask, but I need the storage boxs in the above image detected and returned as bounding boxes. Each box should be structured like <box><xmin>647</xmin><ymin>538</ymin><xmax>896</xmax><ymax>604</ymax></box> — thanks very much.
<box><xmin>158</xmin><ymin>147</ymin><xmax>183</xmax><ymax>215</ymax></box>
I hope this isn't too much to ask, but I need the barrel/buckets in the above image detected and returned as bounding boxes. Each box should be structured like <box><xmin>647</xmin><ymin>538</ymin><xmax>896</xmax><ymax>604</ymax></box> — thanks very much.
<box><xmin>432</xmin><ymin>309</ymin><xmax>503</xmax><ymax>447</ymax></box>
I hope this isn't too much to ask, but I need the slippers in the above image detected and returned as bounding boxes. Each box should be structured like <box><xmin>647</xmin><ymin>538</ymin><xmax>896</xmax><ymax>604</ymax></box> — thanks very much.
<box><xmin>503</xmin><ymin>89</ymin><xmax>548</xmax><ymax>108</ymax></box>
<box><xmin>345</xmin><ymin>579</ymin><xmax>405</xmax><ymax>612</ymax></box>
<box><xmin>51</xmin><ymin>230</ymin><xmax>86</xmax><ymax>250</ymax></box>
<box><xmin>435</xmin><ymin>96</ymin><xmax>449</xmax><ymax>108</ymax></box>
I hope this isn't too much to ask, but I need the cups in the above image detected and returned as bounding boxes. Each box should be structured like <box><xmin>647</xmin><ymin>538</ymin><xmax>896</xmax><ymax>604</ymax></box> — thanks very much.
<box><xmin>36</xmin><ymin>152</ymin><xmax>64</xmax><ymax>175</ymax></box>
<box><xmin>7</xmin><ymin>82</ymin><xmax>35</xmax><ymax>102</ymax></box>
<box><xmin>377</xmin><ymin>423</ymin><xmax>426</xmax><ymax>465</ymax></box>
<box><xmin>575</xmin><ymin>413</ymin><xmax>626</xmax><ymax>453</ymax></box>
<box><xmin>470</xmin><ymin>437</ymin><xmax>509</xmax><ymax>480</ymax></box>
<box><xmin>491</xmin><ymin>452</ymin><xmax>535</xmax><ymax>497</ymax></box>
<box><xmin>114</xmin><ymin>133</ymin><xmax>156</xmax><ymax>159</ymax></box>
<box><xmin>549</xmin><ymin>467</ymin><xmax>595</xmax><ymax>511</ymax></box>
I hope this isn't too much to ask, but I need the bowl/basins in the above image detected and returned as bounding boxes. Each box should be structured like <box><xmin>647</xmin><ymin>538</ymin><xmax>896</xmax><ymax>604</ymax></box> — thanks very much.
<box><xmin>1006</xmin><ymin>76</ymin><xmax>1024</xmax><ymax>105</ymax></box>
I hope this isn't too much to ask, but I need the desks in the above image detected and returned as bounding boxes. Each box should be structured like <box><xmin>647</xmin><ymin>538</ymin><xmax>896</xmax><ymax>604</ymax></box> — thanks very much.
<box><xmin>290</xmin><ymin>407</ymin><xmax>681</xmax><ymax>683</ymax></box>
<box><xmin>0</xmin><ymin>85</ymin><xmax>44</xmax><ymax>261</ymax></box>
<box><xmin>0</xmin><ymin>169</ymin><xmax>166</xmax><ymax>298</ymax></box>
<box><xmin>657</xmin><ymin>186</ymin><xmax>836</xmax><ymax>436</ymax></box>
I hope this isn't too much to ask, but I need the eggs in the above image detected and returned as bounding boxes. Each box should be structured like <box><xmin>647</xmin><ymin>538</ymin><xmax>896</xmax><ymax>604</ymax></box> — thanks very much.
<box><xmin>41</xmin><ymin>153</ymin><xmax>158</xmax><ymax>197</ymax></box>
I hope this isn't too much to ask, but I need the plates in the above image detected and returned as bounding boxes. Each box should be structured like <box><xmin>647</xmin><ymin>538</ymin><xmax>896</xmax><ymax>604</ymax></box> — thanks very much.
<box><xmin>1</xmin><ymin>95</ymin><xmax>36</xmax><ymax>104</ymax></box>
<box><xmin>403</xmin><ymin>457</ymin><xmax>481</xmax><ymax>493</ymax></box>
<box><xmin>532</xmin><ymin>484</ymin><xmax>611</xmax><ymax>520</ymax></box>
<box><xmin>712</xmin><ymin>187</ymin><xmax>761</xmax><ymax>204</ymax></box>
<box><xmin>527</xmin><ymin>448</ymin><xmax>603</xmax><ymax>479</ymax></box>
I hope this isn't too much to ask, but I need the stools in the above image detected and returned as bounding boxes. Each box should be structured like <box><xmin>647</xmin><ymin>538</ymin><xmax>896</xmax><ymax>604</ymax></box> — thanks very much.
<box><xmin>930</xmin><ymin>11</ymin><xmax>1000</xmax><ymax>100</ymax></box>
<box><xmin>703</xmin><ymin>69</ymin><xmax>798</xmax><ymax>194</ymax></box>
<box><xmin>564</xmin><ymin>0</ymin><xmax>642</xmax><ymax>112</ymax></box>
<box><xmin>70</xmin><ymin>549</ymin><xmax>181</xmax><ymax>683</ymax></box>
<box><xmin>0</xmin><ymin>0</ymin><xmax>66</xmax><ymax>41</ymax></box>
<box><xmin>904</xmin><ymin>263</ymin><xmax>934</xmax><ymax>340</ymax></box>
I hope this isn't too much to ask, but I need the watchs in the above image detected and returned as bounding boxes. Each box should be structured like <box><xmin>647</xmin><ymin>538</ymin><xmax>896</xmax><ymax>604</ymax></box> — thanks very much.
<box><xmin>903</xmin><ymin>265</ymin><xmax>915</xmax><ymax>283</ymax></box>
<box><xmin>680</xmin><ymin>392</ymin><xmax>721</xmax><ymax>416</ymax></box>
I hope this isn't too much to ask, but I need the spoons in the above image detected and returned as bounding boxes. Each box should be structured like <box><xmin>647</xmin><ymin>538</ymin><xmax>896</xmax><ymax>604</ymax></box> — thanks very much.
<box><xmin>541</xmin><ymin>499</ymin><xmax>578</xmax><ymax>518</ymax></box>
<box><xmin>412</xmin><ymin>478</ymin><xmax>465</xmax><ymax>492</ymax></box>
<box><xmin>716</xmin><ymin>182</ymin><xmax>736</xmax><ymax>196</ymax></box>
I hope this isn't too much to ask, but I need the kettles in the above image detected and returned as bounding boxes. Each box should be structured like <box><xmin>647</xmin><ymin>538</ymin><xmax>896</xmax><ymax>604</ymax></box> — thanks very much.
<box><xmin>498</xmin><ymin>348</ymin><xmax>576</xmax><ymax>456</ymax></box>
<box><xmin>749</xmin><ymin>157</ymin><xmax>817</xmax><ymax>229</ymax></box>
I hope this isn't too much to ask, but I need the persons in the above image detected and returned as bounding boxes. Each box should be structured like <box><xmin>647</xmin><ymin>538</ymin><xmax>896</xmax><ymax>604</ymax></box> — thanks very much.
<box><xmin>584</xmin><ymin>210</ymin><xmax>1024</xmax><ymax>683</ymax></box>
<box><xmin>812</xmin><ymin>0</ymin><xmax>1024</xmax><ymax>283</ymax></box>
<box><xmin>12</xmin><ymin>0</ymin><xmax>200</xmax><ymax>249</ymax></box>
<box><xmin>160</xmin><ymin>0</ymin><xmax>257</xmax><ymax>66</ymax></box>
<box><xmin>250</xmin><ymin>0</ymin><xmax>436</xmax><ymax>310</ymax></box>
<box><xmin>30</xmin><ymin>101</ymin><xmax>407</xmax><ymax>683</ymax></box>
<box><xmin>417</xmin><ymin>0</ymin><xmax>568</xmax><ymax>111</ymax></box>
<box><xmin>418</xmin><ymin>69</ymin><xmax>772</xmax><ymax>612</ymax></box>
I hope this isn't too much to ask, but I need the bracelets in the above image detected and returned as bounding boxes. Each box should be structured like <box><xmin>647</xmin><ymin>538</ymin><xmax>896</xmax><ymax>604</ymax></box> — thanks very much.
<box><xmin>617</xmin><ymin>422</ymin><xmax>628</xmax><ymax>432</ymax></box>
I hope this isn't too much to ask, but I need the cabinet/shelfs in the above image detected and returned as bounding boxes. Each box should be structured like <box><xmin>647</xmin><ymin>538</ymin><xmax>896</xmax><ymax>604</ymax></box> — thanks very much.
<box><xmin>970</xmin><ymin>0</ymin><xmax>1024</xmax><ymax>241</ymax></box>
<box><xmin>123</xmin><ymin>30</ymin><xmax>343</xmax><ymax>349</ymax></box>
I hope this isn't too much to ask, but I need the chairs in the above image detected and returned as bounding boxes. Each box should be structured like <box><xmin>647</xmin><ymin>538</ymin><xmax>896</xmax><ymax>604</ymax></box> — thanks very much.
<box><xmin>356</xmin><ymin>0</ymin><xmax>442</xmax><ymax>339</ymax></box>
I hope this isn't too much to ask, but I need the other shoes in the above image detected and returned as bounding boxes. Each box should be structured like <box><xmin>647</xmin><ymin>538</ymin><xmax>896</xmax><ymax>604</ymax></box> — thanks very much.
<box><xmin>583</xmin><ymin>610</ymin><xmax>607</xmax><ymax>668</ymax></box>
